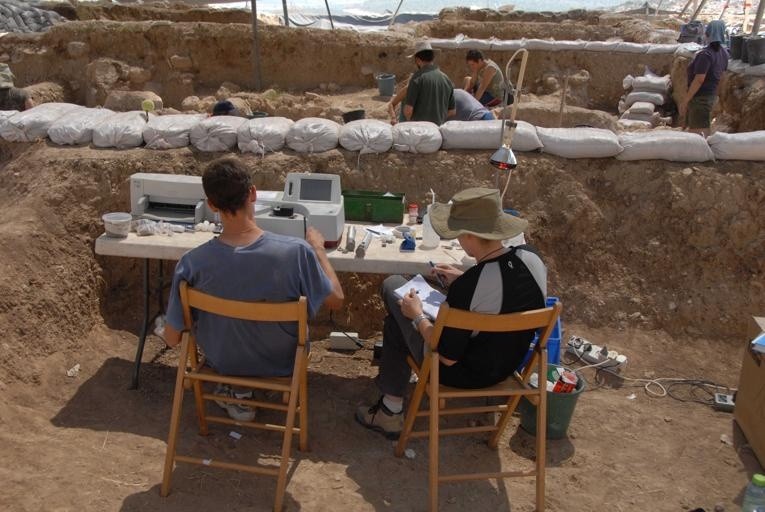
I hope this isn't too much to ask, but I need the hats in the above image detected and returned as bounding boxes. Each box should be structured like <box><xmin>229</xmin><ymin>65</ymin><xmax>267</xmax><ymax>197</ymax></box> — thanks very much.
<box><xmin>429</xmin><ymin>187</ymin><xmax>528</xmax><ymax>240</ymax></box>
<box><xmin>406</xmin><ymin>41</ymin><xmax>441</xmax><ymax>58</ymax></box>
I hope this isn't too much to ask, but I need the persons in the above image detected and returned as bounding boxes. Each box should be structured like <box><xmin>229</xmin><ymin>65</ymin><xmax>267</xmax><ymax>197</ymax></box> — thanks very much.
<box><xmin>448</xmin><ymin>88</ymin><xmax>495</xmax><ymax>120</ymax></box>
<box><xmin>679</xmin><ymin>19</ymin><xmax>728</xmax><ymax>138</ymax></box>
<box><xmin>402</xmin><ymin>40</ymin><xmax>456</xmax><ymax>127</ymax></box>
<box><xmin>462</xmin><ymin>49</ymin><xmax>505</xmax><ymax>106</ymax></box>
<box><xmin>0</xmin><ymin>51</ymin><xmax>37</xmax><ymax>111</ymax></box>
<box><xmin>352</xmin><ymin>187</ymin><xmax>548</xmax><ymax>440</ymax></box>
<box><xmin>388</xmin><ymin>85</ymin><xmax>408</xmax><ymax>126</ymax></box>
<box><xmin>163</xmin><ymin>157</ymin><xmax>344</xmax><ymax>422</ymax></box>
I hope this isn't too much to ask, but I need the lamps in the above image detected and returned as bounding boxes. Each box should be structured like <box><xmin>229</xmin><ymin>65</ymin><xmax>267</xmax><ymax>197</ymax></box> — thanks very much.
<box><xmin>491</xmin><ymin>46</ymin><xmax>534</xmax><ymax>202</ymax></box>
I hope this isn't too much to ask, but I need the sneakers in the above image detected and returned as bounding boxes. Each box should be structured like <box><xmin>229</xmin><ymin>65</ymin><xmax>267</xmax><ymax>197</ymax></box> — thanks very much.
<box><xmin>213</xmin><ymin>383</ymin><xmax>256</xmax><ymax>421</ymax></box>
<box><xmin>152</xmin><ymin>313</ymin><xmax>166</xmax><ymax>342</ymax></box>
<box><xmin>356</xmin><ymin>395</ymin><xmax>404</xmax><ymax>441</ymax></box>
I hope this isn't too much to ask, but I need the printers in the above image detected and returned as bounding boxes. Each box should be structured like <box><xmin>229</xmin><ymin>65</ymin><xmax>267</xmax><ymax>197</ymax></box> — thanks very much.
<box><xmin>130</xmin><ymin>173</ymin><xmax>214</xmax><ymax>224</ymax></box>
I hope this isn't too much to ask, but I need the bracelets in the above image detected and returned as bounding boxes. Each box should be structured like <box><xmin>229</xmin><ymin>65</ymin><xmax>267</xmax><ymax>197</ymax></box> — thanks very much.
<box><xmin>413</xmin><ymin>314</ymin><xmax>427</xmax><ymax>330</ymax></box>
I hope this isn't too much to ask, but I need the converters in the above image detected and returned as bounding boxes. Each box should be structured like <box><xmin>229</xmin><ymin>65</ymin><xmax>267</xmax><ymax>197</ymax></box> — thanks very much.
<box><xmin>330</xmin><ymin>332</ymin><xmax>359</xmax><ymax>350</ymax></box>
<box><xmin>615</xmin><ymin>354</ymin><xmax>627</xmax><ymax>367</ymax></box>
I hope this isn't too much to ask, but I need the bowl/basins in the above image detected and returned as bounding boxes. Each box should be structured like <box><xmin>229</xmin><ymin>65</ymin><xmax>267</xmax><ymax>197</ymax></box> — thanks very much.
<box><xmin>101</xmin><ymin>210</ymin><xmax>134</xmax><ymax>239</ymax></box>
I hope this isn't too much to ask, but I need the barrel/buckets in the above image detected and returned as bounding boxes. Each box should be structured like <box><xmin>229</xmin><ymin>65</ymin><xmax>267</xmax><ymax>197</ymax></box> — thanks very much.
<box><xmin>729</xmin><ymin>33</ymin><xmax>765</xmax><ymax>66</ymax></box>
<box><xmin>376</xmin><ymin>73</ymin><xmax>395</xmax><ymax>96</ymax></box>
<box><xmin>247</xmin><ymin>111</ymin><xmax>269</xmax><ymax>119</ymax></box>
<box><xmin>342</xmin><ymin>109</ymin><xmax>365</xmax><ymax>122</ymax></box>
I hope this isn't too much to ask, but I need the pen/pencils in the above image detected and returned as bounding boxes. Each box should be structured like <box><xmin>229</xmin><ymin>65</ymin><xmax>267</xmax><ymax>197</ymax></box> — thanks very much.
<box><xmin>429</xmin><ymin>261</ymin><xmax>445</xmax><ymax>289</ymax></box>
<box><xmin>366</xmin><ymin>228</ymin><xmax>380</xmax><ymax>235</ymax></box>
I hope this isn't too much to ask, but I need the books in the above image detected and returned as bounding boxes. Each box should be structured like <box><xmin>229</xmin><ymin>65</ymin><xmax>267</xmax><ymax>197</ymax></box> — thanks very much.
<box><xmin>395</xmin><ymin>274</ymin><xmax>446</xmax><ymax>320</ymax></box>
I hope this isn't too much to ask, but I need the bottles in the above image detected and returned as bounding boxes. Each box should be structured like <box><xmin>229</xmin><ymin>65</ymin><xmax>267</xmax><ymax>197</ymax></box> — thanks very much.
<box><xmin>741</xmin><ymin>472</ymin><xmax>765</xmax><ymax>512</ymax></box>
<box><xmin>422</xmin><ymin>204</ymin><xmax>441</xmax><ymax>249</ymax></box>
<box><xmin>490</xmin><ymin>120</ymin><xmax>518</xmax><ymax>170</ymax></box>
<box><xmin>408</xmin><ymin>204</ymin><xmax>420</xmax><ymax>226</ymax></box>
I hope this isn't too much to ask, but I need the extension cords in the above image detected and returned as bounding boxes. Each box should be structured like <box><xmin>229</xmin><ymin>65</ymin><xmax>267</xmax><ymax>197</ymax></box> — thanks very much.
<box><xmin>714</xmin><ymin>393</ymin><xmax>736</xmax><ymax>413</ymax></box>
<box><xmin>566</xmin><ymin>335</ymin><xmax>628</xmax><ymax>374</ymax></box>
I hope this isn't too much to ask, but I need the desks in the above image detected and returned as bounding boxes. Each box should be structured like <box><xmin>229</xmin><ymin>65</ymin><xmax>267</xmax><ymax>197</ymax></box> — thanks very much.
<box><xmin>94</xmin><ymin>203</ymin><xmax>525</xmax><ymax>393</ymax></box>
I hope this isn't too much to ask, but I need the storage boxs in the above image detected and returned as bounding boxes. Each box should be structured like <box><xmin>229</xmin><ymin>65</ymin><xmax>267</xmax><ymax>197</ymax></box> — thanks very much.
<box><xmin>521</xmin><ymin>295</ymin><xmax>563</xmax><ymax>374</ymax></box>
<box><xmin>733</xmin><ymin>313</ymin><xmax>764</xmax><ymax>471</ymax></box>
<box><xmin>337</xmin><ymin>188</ymin><xmax>406</xmax><ymax>224</ymax></box>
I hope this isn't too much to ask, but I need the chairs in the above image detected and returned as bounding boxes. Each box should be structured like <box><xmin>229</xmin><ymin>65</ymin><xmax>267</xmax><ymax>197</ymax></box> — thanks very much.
<box><xmin>159</xmin><ymin>281</ymin><xmax>309</xmax><ymax>511</ymax></box>
<box><xmin>394</xmin><ymin>301</ymin><xmax>563</xmax><ymax>512</ymax></box>
